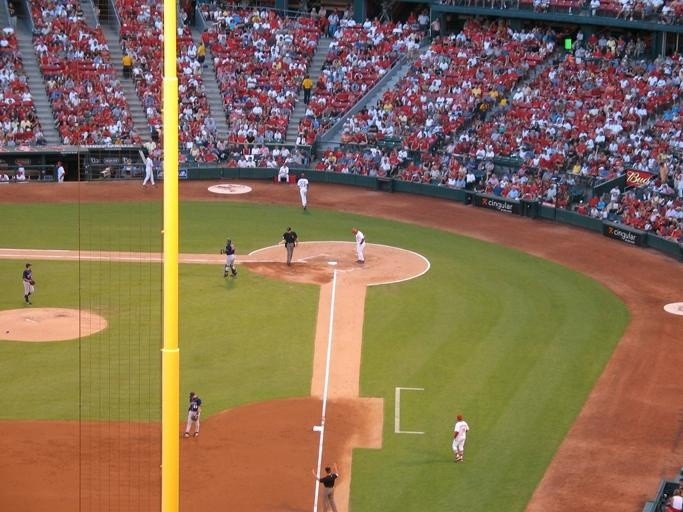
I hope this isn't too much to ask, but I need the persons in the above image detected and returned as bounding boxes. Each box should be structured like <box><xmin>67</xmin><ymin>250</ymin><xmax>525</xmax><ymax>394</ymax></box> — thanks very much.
<box><xmin>278</xmin><ymin>226</ymin><xmax>298</xmax><ymax>266</ymax></box>
<box><xmin>219</xmin><ymin>237</ymin><xmax>238</xmax><ymax>279</ymax></box>
<box><xmin>21</xmin><ymin>262</ymin><xmax>35</xmax><ymax>305</ymax></box>
<box><xmin>350</xmin><ymin>226</ymin><xmax>367</xmax><ymax>264</ymax></box>
<box><xmin>310</xmin><ymin>461</ymin><xmax>340</xmax><ymax>512</ymax></box>
<box><xmin>390</xmin><ymin>1</ymin><xmax>682</xmax><ymax>245</ymax></box>
<box><xmin>659</xmin><ymin>467</ymin><xmax>682</xmax><ymax>512</ymax></box>
<box><xmin>182</xmin><ymin>391</ymin><xmax>202</xmax><ymax>439</ymax></box>
<box><xmin>450</xmin><ymin>414</ymin><xmax>470</xmax><ymax>463</ymax></box>
<box><xmin>0</xmin><ymin>1</ymin><xmax>389</xmax><ymax>183</ymax></box>
<box><xmin>296</xmin><ymin>172</ymin><xmax>310</xmax><ymax>211</ymax></box>
<box><xmin>140</xmin><ymin>154</ymin><xmax>156</xmax><ymax>189</ymax></box>
